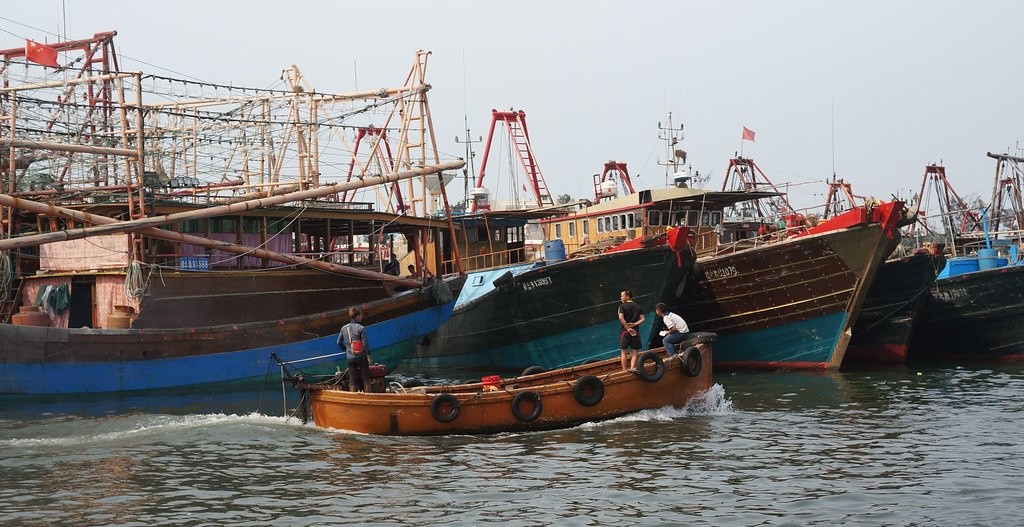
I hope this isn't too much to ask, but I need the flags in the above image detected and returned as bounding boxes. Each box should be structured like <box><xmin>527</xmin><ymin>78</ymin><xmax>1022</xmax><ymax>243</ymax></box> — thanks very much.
<box><xmin>27</xmin><ymin>39</ymin><xmax>58</xmax><ymax>70</ymax></box>
<box><xmin>743</xmin><ymin>127</ymin><xmax>756</xmax><ymax>143</ymax></box>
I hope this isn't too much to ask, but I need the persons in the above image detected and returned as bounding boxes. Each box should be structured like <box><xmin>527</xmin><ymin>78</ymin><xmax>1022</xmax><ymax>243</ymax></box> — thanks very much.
<box><xmin>337</xmin><ymin>308</ymin><xmax>372</xmax><ymax>393</ymax></box>
<box><xmin>391</xmin><ymin>254</ymin><xmax>400</xmax><ymax>276</ymax></box>
<box><xmin>656</xmin><ymin>303</ymin><xmax>689</xmax><ymax>355</ymax></box>
<box><xmin>408</xmin><ymin>265</ymin><xmax>416</xmax><ymax>278</ymax></box>
<box><xmin>618</xmin><ymin>290</ymin><xmax>645</xmax><ymax>370</ymax></box>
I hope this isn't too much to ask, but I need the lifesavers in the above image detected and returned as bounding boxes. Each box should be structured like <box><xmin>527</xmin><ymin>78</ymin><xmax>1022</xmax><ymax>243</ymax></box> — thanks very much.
<box><xmin>635</xmin><ymin>350</ymin><xmax>667</xmax><ymax>383</ymax></box>
<box><xmin>512</xmin><ymin>389</ymin><xmax>542</xmax><ymax>424</ymax></box>
<box><xmin>681</xmin><ymin>346</ymin><xmax>703</xmax><ymax>378</ymax></box>
<box><xmin>430</xmin><ymin>392</ymin><xmax>461</xmax><ymax>424</ymax></box>
<box><xmin>572</xmin><ymin>373</ymin><xmax>606</xmax><ymax>409</ymax></box>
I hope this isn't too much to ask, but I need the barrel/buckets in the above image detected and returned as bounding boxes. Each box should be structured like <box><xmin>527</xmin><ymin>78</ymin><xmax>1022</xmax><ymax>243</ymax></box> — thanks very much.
<box><xmin>978</xmin><ymin>249</ymin><xmax>998</xmax><ymax>270</ymax></box>
<box><xmin>482</xmin><ymin>375</ymin><xmax>500</xmax><ymax>391</ymax></box>
<box><xmin>949</xmin><ymin>256</ymin><xmax>979</xmax><ymax>276</ymax></box>
<box><xmin>544</xmin><ymin>239</ymin><xmax>566</xmax><ymax>265</ymax></box>
<box><xmin>997</xmin><ymin>258</ymin><xmax>1007</xmax><ymax>267</ymax></box>
<box><xmin>938</xmin><ymin>259</ymin><xmax>949</xmax><ymax>279</ymax></box>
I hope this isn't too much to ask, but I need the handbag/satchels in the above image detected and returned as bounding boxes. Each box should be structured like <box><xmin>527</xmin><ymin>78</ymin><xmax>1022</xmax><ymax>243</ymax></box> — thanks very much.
<box><xmin>347</xmin><ymin>324</ymin><xmax>364</xmax><ymax>355</ymax></box>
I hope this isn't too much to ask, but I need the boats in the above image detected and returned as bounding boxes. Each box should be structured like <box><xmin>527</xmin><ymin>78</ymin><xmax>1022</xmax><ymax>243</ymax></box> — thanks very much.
<box><xmin>1</xmin><ymin>2</ymin><xmax>471</xmax><ymax>416</ymax></box>
<box><xmin>405</xmin><ymin>111</ymin><xmax>1023</xmax><ymax>382</ymax></box>
<box><xmin>265</xmin><ymin>331</ymin><xmax>718</xmax><ymax>437</ymax></box>
